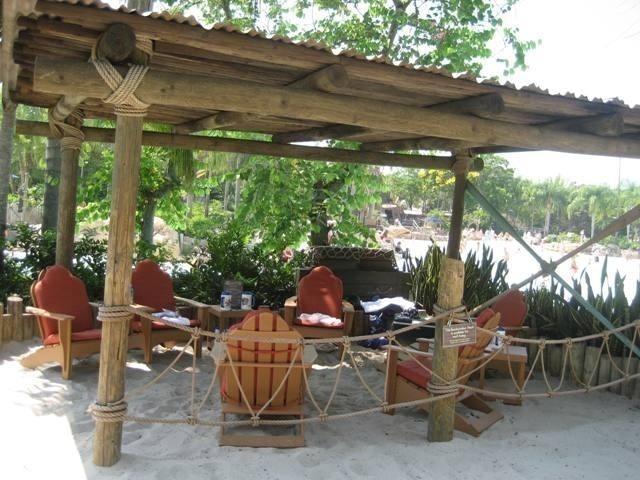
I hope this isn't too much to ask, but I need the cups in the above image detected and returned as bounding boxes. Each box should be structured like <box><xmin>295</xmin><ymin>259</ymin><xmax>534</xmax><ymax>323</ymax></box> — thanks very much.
<box><xmin>240</xmin><ymin>291</ymin><xmax>255</xmax><ymax>310</ymax></box>
<box><xmin>495</xmin><ymin>328</ymin><xmax>506</xmax><ymax>347</ymax></box>
<box><xmin>219</xmin><ymin>291</ymin><xmax>231</xmax><ymax>311</ymax></box>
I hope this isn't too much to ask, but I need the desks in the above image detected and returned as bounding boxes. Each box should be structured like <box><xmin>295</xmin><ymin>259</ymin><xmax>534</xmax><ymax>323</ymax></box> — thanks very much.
<box><xmin>208</xmin><ymin>304</ymin><xmax>255</xmax><ymax>351</ymax></box>
<box><xmin>478</xmin><ymin>345</ymin><xmax>526</xmax><ymax>393</ymax></box>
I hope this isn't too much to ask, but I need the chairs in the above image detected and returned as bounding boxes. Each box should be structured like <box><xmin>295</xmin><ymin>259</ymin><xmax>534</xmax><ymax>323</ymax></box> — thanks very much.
<box><xmin>479</xmin><ymin>288</ymin><xmax>529</xmax><ymax>345</ymax></box>
<box><xmin>19</xmin><ymin>265</ymin><xmax>134</xmax><ymax>380</ymax></box>
<box><xmin>215</xmin><ymin>310</ymin><xmax>311</xmax><ymax>449</ymax></box>
<box><xmin>383</xmin><ymin>309</ymin><xmax>503</xmax><ymax>436</ymax></box>
<box><xmin>283</xmin><ymin>266</ymin><xmax>355</xmax><ymax>362</ymax></box>
<box><xmin>133</xmin><ymin>258</ymin><xmax>209</xmax><ymax>365</ymax></box>
